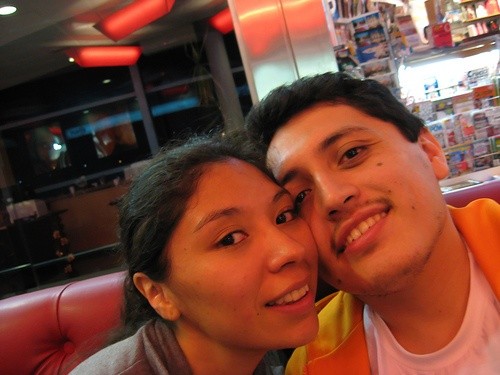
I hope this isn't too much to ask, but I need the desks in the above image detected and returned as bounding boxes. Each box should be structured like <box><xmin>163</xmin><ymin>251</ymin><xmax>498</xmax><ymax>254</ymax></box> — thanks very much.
<box><xmin>0</xmin><ymin>206</ymin><xmax>77</xmax><ymax>288</ymax></box>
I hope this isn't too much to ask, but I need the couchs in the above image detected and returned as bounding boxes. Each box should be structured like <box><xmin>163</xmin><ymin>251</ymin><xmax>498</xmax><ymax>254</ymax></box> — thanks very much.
<box><xmin>0</xmin><ymin>270</ymin><xmax>128</xmax><ymax>375</ymax></box>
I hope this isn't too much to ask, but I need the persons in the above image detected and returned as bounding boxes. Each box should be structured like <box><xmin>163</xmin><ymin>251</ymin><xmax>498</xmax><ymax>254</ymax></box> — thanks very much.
<box><xmin>235</xmin><ymin>70</ymin><xmax>500</xmax><ymax>375</ymax></box>
<box><xmin>64</xmin><ymin>133</ymin><xmax>321</xmax><ymax>375</ymax></box>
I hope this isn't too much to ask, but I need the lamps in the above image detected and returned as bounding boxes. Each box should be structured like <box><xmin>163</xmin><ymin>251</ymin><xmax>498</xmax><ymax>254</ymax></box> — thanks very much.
<box><xmin>209</xmin><ymin>6</ymin><xmax>235</xmax><ymax>35</ymax></box>
<box><xmin>62</xmin><ymin>45</ymin><xmax>143</xmax><ymax>68</ymax></box>
<box><xmin>93</xmin><ymin>0</ymin><xmax>175</xmax><ymax>43</ymax></box>
